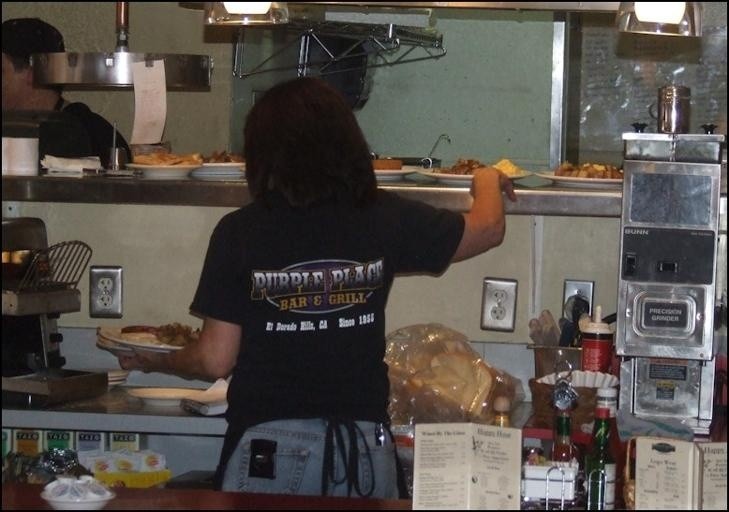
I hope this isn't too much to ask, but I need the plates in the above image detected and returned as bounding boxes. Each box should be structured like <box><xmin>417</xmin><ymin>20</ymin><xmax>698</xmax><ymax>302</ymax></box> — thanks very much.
<box><xmin>126</xmin><ymin>387</ymin><xmax>205</xmax><ymax>409</ymax></box>
<box><xmin>421</xmin><ymin>169</ymin><xmax>533</xmax><ymax>187</ymax></box>
<box><xmin>125</xmin><ymin>163</ymin><xmax>203</xmax><ymax>181</ymax></box>
<box><xmin>95</xmin><ymin>327</ymin><xmax>189</xmax><ymax>352</ymax></box>
<box><xmin>192</xmin><ymin>162</ymin><xmax>247</xmax><ymax>181</ymax></box>
<box><xmin>536</xmin><ymin>171</ymin><xmax>626</xmax><ymax>190</ymax></box>
<box><xmin>373</xmin><ymin>168</ymin><xmax>417</xmax><ymax>183</ymax></box>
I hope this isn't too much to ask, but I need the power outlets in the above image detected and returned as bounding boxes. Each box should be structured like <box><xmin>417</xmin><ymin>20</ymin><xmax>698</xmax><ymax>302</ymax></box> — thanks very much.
<box><xmin>480</xmin><ymin>278</ymin><xmax>518</xmax><ymax>332</ymax></box>
<box><xmin>89</xmin><ymin>266</ymin><xmax>122</xmax><ymax>317</ymax></box>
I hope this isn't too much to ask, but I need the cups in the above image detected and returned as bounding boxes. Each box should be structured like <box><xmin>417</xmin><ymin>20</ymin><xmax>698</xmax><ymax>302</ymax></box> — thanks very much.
<box><xmin>1</xmin><ymin>138</ymin><xmax>39</xmax><ymax>178</ymax></box>
<box><xmin>650</xmin><ymin>86</ymin><xmax>690</xmax><ymax>134</ymax></box>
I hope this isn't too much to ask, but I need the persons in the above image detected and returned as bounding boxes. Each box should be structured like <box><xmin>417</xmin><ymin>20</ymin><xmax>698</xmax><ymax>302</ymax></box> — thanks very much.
<box><xmin>116</xmin><ymin>76</ymin><xmax>516</xmax><ymax>498</ymax></box>
<box><xmin>0</xmin><ymin>17</ymin><xmax>133</xmax><ymax>166</ymax></box>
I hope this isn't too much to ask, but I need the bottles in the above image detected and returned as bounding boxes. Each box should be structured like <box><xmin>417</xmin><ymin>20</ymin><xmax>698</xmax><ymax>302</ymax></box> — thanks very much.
<box><xmin>580</xmin><ymin>304</ymin><xmax>614</xmax><ymax>374</ymax></box>
<box><xmin>492</xmin><ymin>397</ymin><xmax>511</xmax><ymax>427</ymax></box>
<box><xmin>555</xmin><ymin>387</ymin><xmax>620</xmax><ymax>511</ymax></box>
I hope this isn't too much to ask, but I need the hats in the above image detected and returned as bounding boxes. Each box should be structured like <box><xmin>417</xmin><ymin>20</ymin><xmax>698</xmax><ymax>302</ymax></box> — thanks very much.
<box><xmin>0</xmin><ymin>18</ymin><xmax>65</xmax><ymax>54</ymax></box>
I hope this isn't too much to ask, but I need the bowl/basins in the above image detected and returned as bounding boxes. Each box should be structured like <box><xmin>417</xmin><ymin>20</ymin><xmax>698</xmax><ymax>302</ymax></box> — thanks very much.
<box><xmin>40</xmin><ymin>492</ymin><xmax>116</xmax><ymax>512</ymax></box>
